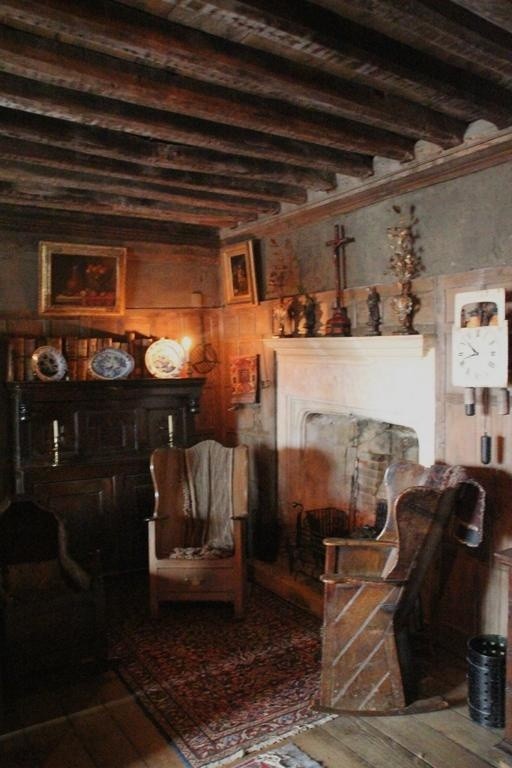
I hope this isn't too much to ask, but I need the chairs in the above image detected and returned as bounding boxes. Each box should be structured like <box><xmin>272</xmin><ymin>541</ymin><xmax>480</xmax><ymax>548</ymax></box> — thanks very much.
<box><xmin>317</xmin><ymin>461</ymin><xmax>469</xmax><ymax>711</ymax></box>
<box><xmin>144</xmin><ymin>439</ymin><xmax>250</xmax><ymax>621</ymax></box>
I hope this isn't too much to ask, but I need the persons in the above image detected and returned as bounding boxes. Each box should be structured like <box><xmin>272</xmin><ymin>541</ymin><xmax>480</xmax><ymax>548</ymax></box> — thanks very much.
<box><xmin>281</xmin><ymin>296</ymin><xmax>298</xmax><ymax>336</ymax></box>
<box><xmin>296</xmin><ymin>294</ymin><xmax>315</xmax><ymax>336</ymax></box>
<box><xmin>364</xmin><ymin>286</ymin><xmax>381</xmax><ymax>336</ymax></box>
<box><xmin>234</xmin><ymin>262</ymin><xmax>248</xmax><ymax>295</ymax></box>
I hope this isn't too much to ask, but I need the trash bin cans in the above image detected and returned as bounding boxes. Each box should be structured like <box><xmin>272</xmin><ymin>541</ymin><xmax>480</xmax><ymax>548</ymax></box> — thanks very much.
<box><xmin>465</xmin><ymin>633</ymin><xmax>507</xmax><ymax>728</ymax></box>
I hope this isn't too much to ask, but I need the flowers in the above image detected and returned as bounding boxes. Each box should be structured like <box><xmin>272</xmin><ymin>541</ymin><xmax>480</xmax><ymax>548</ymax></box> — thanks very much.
<box><xmin>383</xmin><ymin>203</ymin><xmax>428</xmax><ymax>281</ymax></box>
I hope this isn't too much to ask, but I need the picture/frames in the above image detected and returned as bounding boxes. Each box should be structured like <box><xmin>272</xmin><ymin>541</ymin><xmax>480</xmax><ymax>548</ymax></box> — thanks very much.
<box><xmin>219</xmin><ymin>239</ymin><xmax>258</xmax><ymax>310</ymax></box>
<box><xmin>37</xmin><ymin>240</ymin><xmax>127</xmax><ymax>316</ymax></box>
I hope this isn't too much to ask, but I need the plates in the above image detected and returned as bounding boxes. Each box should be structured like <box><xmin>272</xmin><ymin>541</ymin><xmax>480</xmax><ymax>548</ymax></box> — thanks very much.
<box><xmin>31</xmin><ymin>345</ymin><xmax>68</xmax><ymax>383</ymax></box>
<box><xmin>87</xmin><ymin>347</ymin><xmax>135</xmax><ymax>380</ymax></box>
<box><xmin>144</xmin><ymin>339</ymin><xmax>186</xmax><ymax>379</ymax></box>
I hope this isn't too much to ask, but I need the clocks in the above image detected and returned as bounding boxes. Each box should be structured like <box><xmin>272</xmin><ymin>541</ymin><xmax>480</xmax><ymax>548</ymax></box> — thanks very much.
<box><xmin>450</xmin><ymin>288</ymin><xmax>510</xmax><ymax>388</ymax></box>
